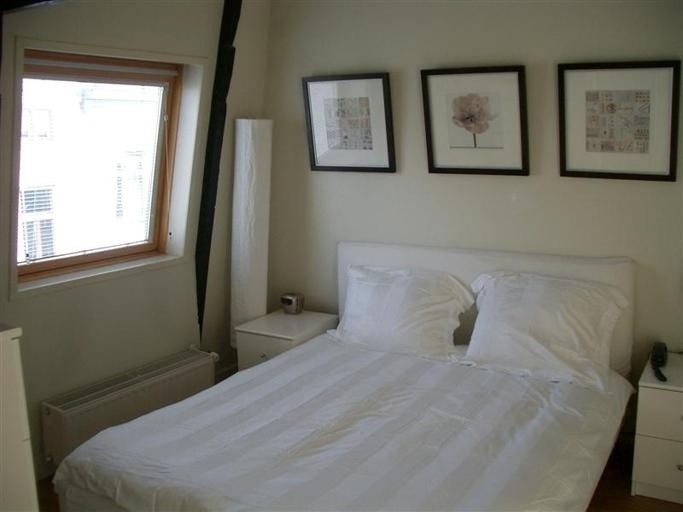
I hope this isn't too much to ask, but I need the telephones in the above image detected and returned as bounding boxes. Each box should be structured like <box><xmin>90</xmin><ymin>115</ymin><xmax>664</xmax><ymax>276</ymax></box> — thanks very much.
<box><xmin>650</xmin><ymin>342</ymin><xmax>668</xmax><ymax>367</ymax></box>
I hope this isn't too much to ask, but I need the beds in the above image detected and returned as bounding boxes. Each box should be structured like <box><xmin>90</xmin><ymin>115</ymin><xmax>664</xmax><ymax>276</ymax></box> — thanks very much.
<box><xmin>52</xmin><ymin>242</ymin><xmax>636</xmax><ymax>511</ymax></box>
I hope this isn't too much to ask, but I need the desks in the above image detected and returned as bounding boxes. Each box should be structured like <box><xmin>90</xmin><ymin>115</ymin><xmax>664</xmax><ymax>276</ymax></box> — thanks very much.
<box><xmin>0</xmin><ymin>324</ymin><xmax>41</xmax><ymax>512</ymax></box>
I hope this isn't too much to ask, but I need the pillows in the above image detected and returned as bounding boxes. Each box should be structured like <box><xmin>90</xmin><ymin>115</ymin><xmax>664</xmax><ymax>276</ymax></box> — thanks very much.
<box><xmin>461</xmin><ymin>272</ymin><xmax>628</xmax><ymax>393</ymax></box>
<box><xmin>327</xmin><ymin>264</ymin><xmax>475</xmax><ymax>362</ymax></box>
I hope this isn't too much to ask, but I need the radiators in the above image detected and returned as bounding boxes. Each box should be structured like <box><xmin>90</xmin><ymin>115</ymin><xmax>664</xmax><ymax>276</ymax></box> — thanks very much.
<box><xmin>38</xmin><ymin>342</ymin><xmax>220</xmax><ymax>468</ymax></box>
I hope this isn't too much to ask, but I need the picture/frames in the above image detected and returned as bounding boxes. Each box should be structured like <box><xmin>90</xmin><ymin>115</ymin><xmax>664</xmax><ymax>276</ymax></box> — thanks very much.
<box><xmin>558</xmin><ymin>60</ymin><xmax>680</xmax><ymax>182</ymax></box>
<box><xmin>421</xmin><ymin>65</ymin><xmax>530</xmax><ymax>176</ymax></box>
<box><xmin>302</xmin><ymin>72</ymin><xmax>396</xmax><ymax>174</ymax></box>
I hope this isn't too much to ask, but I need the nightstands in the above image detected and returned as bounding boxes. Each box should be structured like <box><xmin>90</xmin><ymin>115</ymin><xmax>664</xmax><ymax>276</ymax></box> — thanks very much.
<box><xmin>631</xmin><ymin>352</ymin><xmax>682</xmax><ymax>504</ymax></box>
<box><xmin>234</xmin><ymin>309</ymin><xmax>338</xmax><ymax>371</ymax></box>
